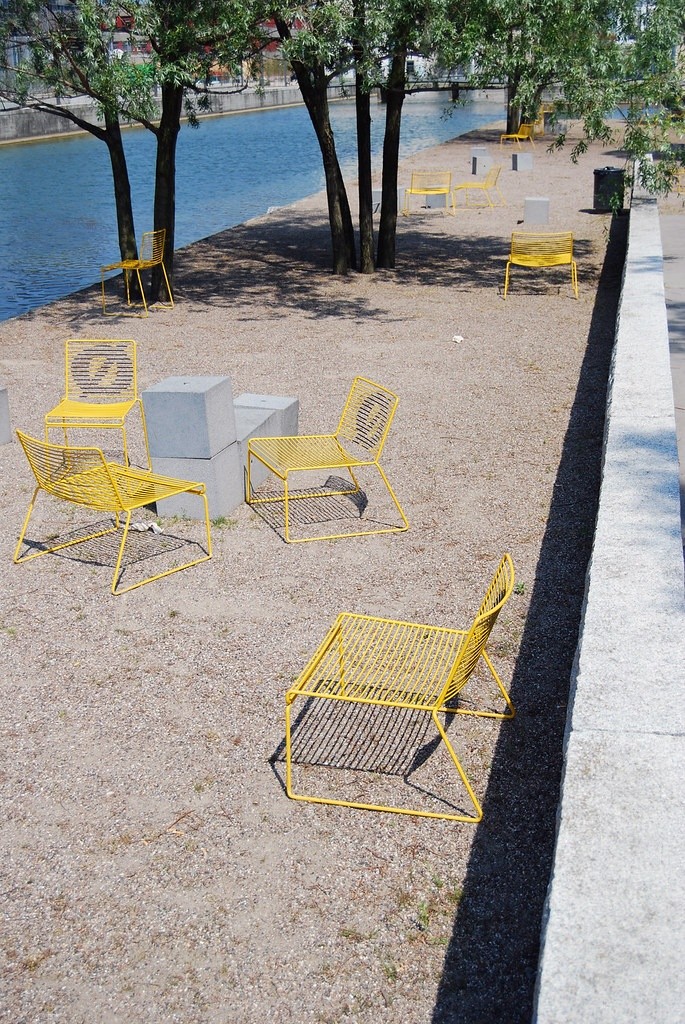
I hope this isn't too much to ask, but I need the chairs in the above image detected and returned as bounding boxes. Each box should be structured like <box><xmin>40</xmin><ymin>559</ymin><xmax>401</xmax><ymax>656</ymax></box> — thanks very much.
<box><xmin>503</xmin><ymin>232</ymin><xmax>579</xmax><ymax>300</ymax></box>
<box><xmin>284</xmin><ymin>553</ymin><xmax>515</xmax><ymax>822</ymax></box>
<box><xmin>500</xmin><ymin>124</ymin><xmax>536</xmax><ymax>151</ymax></box>
<box><xmin>534</xmin><ymin>104</ymin><xmax>544</xmax><ymax>137</ymax></box>
<box><xmin>43</xmin><ymin>339</ymin><xmax>152</xmax><ymax>498</ymax></box>
<box><xmin>454</xmin><ymin>166</ymin><xmax>507</xmax><ymax>208</ymax></box>
<box><xmin>246</xmin><ymin>376</ymin><xmax>408</xmax><ymax>543</ymax></box>
<box><xmin>14</xmin><ymin>430</ymin><xmax>212</xmax><ymax>596</ymax></box>
<box><xmin>401</xmin><ymin>171</ymin><xmax>456</xmax><ymax>217</ymax></box>
<box><xmin>101</xmin><ymin>229</ymin><xmax>174</xmax><ymax>318</ymax></box>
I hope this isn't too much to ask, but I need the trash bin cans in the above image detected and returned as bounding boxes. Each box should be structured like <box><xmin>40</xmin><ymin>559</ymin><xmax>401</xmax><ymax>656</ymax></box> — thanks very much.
<box><xmin>593</xmin><ymin>166</ymin><xmax>626</xmax><ymax>212</ymax></box>
